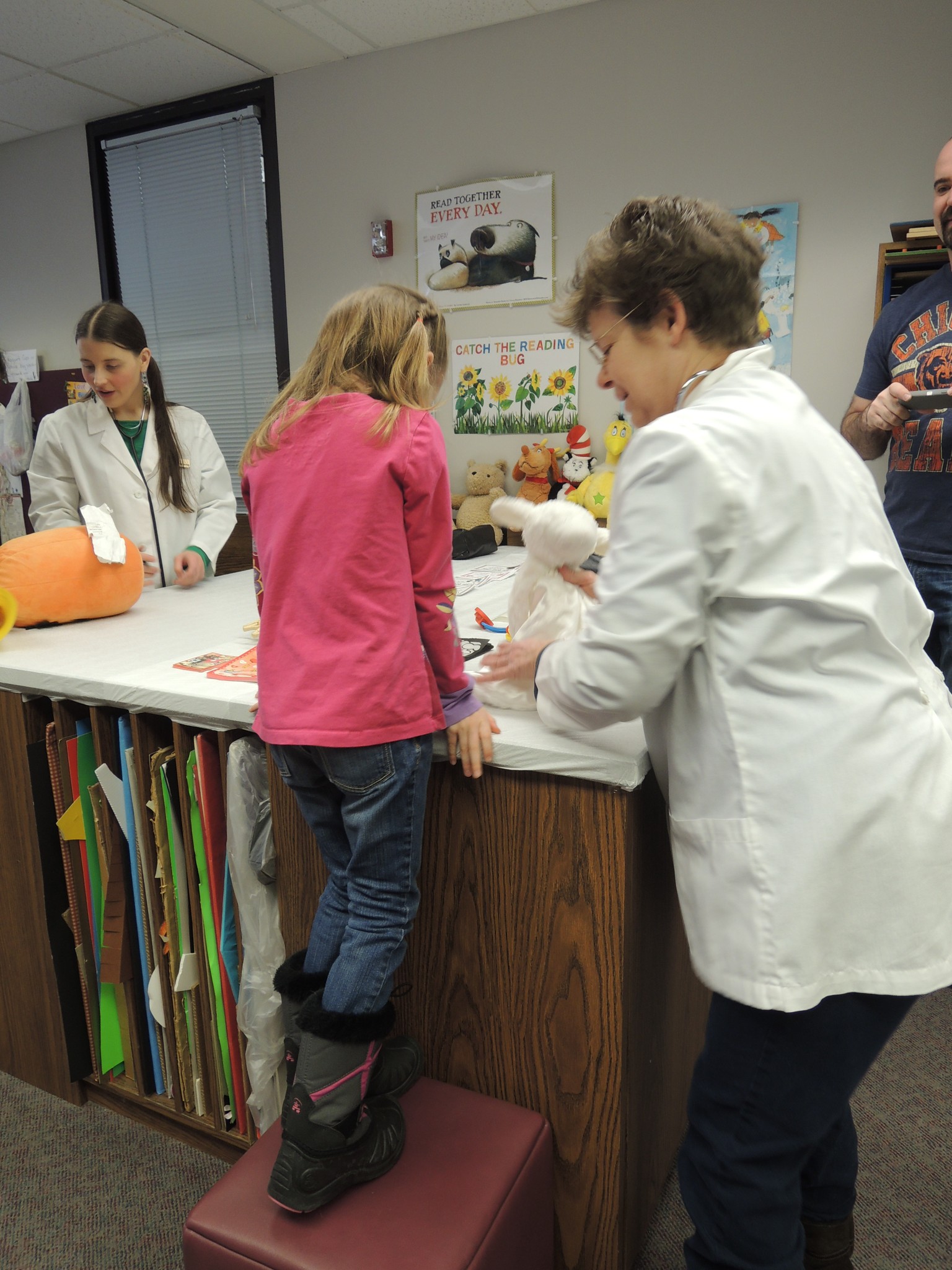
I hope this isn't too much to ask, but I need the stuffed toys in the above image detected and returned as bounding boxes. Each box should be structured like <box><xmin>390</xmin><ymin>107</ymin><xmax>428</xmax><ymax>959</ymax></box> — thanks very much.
<box><xmin>567</xmin><ymin>413</ymin><xmax>633</xmax><ymax>529</ymax></box>
<box><xmin>489</xmin><ymin>496</ymin><xmax>611</xmax><ymax>637</ymax></box>
<box><xmin>548</xmin><ymin>425</ymin><xmax>597</xmax><ymax>500</ymax></box>
<box><xmin>450</xmin><ymin>459</ymin><xmax>508</xmax><ymax>545</ymax></box>
<box><xmin>512</xmin><ymin>438</ymin><xmax>569</xmax><ymax>546</ymax></box>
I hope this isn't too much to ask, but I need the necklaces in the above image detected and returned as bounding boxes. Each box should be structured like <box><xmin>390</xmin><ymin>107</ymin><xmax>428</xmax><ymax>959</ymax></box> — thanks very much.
<box><xmin>108</xmin><ymin>403</ymin><xmax>165</xmax><ymax>587</ymax></box>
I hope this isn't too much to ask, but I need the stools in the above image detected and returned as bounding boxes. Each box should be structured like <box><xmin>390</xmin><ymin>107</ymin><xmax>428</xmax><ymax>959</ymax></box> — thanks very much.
<box><xmin>181</xmin><ymin>1078</ymin><xmax>559</xmax><ymax>1270</ymax></box>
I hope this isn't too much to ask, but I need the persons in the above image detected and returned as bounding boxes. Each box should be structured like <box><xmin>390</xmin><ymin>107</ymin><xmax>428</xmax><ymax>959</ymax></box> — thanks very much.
<box><xmin>238</xmin><ymin>286</ymin><xmax>500</xmax><ymax>1215</ymax></box>
<box><xmin>472</xmin><ymin>193</ymin><xmax>952</xmax><ymax>1270</ymax></box>
<box><xmin>23</xmin><ymin>302</ymin><xmax>237</xmax><ymax>590</ymax></box>
<box><xmin>840</xmin><ymin>140</ymin><xmax>952</xmax><ymax>689</ymax></box>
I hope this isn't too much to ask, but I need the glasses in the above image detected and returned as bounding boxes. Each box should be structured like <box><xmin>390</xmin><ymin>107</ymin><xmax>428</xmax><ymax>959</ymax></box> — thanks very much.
<box><xmin>588</xmin><ymin>300</ymin><xmax>647</xmax><ymax>365</ymax></box>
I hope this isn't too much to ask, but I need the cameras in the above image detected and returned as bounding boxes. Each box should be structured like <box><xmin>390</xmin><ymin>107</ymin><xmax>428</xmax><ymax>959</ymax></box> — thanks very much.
<box><xmin>899</xmin><ymin>388</ymin><xmax>951</xmax><ymax>410</ymax></box>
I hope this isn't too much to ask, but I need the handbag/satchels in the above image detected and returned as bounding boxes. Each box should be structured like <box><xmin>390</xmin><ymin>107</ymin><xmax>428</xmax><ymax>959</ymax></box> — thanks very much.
<box><xmin>0</xmin><ymin>381</ymin><xmax>33</xmax><ymax>476</ymax></box>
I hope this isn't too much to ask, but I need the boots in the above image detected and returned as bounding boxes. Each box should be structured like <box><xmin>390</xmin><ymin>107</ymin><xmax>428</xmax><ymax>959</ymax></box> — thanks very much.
<box><xmin>269</xmin><ymin>944</ymin><xmax>420</xmax><ymax>1126</ymax></box>
<box><xmin>264</xmin><ymin>990</ymin><xmax>407</xmax><ymax>1213</ymax></box>
